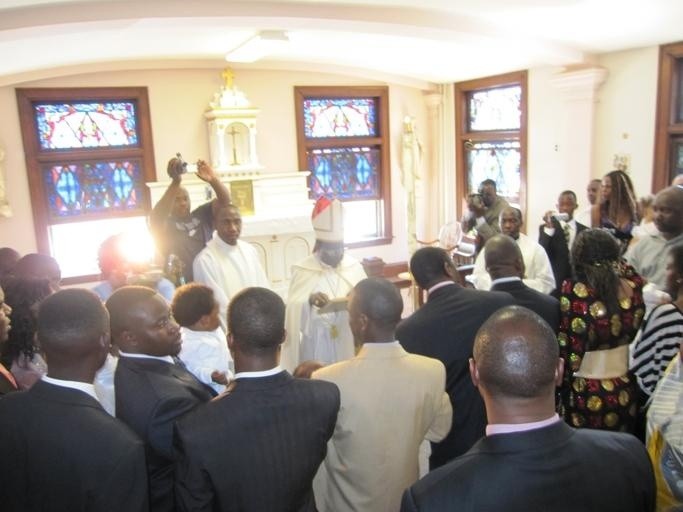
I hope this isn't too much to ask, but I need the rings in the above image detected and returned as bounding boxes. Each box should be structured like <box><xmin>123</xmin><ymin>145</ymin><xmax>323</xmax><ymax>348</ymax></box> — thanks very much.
<box><xmin>315</xmin><ymin>299</ymin><xmax>319</xmax><ymax>305</ymax></box>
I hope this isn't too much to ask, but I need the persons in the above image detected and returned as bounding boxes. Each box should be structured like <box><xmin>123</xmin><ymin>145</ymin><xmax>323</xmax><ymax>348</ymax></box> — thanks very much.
<box><xmin>105</xmin><ymin>285</ymin><xmax>219</xmax><ymax>511</ymax></box>
<box><xmin>308</xmin><ymin>276</ymin><xmax>454</xmax><ymax>512</ymax></box>
<box><xmin>91</xmin><ymin>237</ymin><xmax>177</xmax><ymax>305</ymax></box>
<box><xmin>587</xmin><ymin>179</ymin><xmax>602</xmax><ymax>205</ymax></box>
<box><xmin>623</xmin><ymin>184</ymin><xmax>683</xmax><ymax>290</ymax></box>
<box><xmin>394</xmin><ymin>246</ymin><xmax>517</xmax><ymax>471</ymax></box>
<box><xmin>538</xmin><ymin>191</ymin><xmax>590</xmax><ymax>291</ymax></box>
<box><xmin>1</xmin><ymin>247</ymin><xmax>21</xmax><ymax>290</ymax></box>
<box><xmin>6</xmin><ymin>253</ymin><xmax>61</xmax><ymax>293</ymax></box>
<box><xmin>591</xmin><ymin>170</ymin><xmax>641</xmax><ymax>262</ymax></box>
<box><xmin>0</xmin><ymin>272</ymin><xmax>53</xmax><ymax>391</ymax></box>
<box><xmin>460</xmin><ymin>179</ymin><xmax>510</xmax><ymax>261</ymax></box>
<box><xmin>171</xmin><ymin>282</ymin><xmax>236</xmax><ymax>395</ymax></box>
<box><xmin>555</xmin><ymin>227</ymin><xmax>648</xmax><ymax>444</ymax></box>
<box><xmin>484</xmin><ymin>234</ymin><xmax>563</xmax><ymax>338</ymax></box>
<box><xmin>281</xmin><ymin>194</ymin><xmax>368</xmax><ymax>376</ymax></box>
<box><xmin>629</xmin><ymin>243</ymin><xmax>683</xmax><ymax>445</ymax></box>
<box><xmin>671</xmin><ymin>173</ymin><xmax>683</xmax><ymax>186</ymax></box>
<box><xmin>0</xmin><ymin>288</ymin><xmax>149</xmax><ymax>512</ymax></box>
<box><xmin>192</xmin><ymin>205</ymin><xmax>270</xmax><ymax>331</ymax></box>
<box><xmin>0</xmin><ymin>286</ymin><xmax>20</xmax><ymax>398</ymax></box>
<box><xmin>171</xmin><ymin>287</ymin><xmax>340</xmax><ymax>512</ymax></box>
<box><xmin>149</xmin><ymin>157</ymin><xmax>231</xmax><ymax>288</ymax></box>
<box><xmin>472</xmin><ymin>207</ymin><xmax>557</xmax><ymax>295</ymax></box>
<box><xmin>645</xmin><ymin>338</ymin><xmax>683</xmax><ymax>512</ymax></box>
<box><xmin>401</xmin><ymin>304</ymin><xmax>655</xmax><ymax>512</ymax></box>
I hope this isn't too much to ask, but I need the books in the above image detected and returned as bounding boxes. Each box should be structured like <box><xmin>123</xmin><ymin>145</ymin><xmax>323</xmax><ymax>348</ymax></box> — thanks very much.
<box><xmin>316</xmin><ymin>297</ymin><xmax>349</xmax><ymax>315</ymax></box>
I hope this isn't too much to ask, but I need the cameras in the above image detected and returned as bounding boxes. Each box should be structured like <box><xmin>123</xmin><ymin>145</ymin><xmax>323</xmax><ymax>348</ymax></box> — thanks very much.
<box><xmin>179</xmin><ymin>160</ymin><xmax>198</xmax><ymax>176</ymax></box>
<box><xmin>551</xmin><ymin>212</ymin><xmax>569</xmax><ymax>223</ymax></box>
<box><xmin>468</xmin><ymin>194</ymin><xmax>482</xmax><ymax>205</ymax></box>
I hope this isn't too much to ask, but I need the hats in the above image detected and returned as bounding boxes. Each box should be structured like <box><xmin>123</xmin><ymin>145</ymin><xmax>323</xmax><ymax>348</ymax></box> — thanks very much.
<box><xmin>311</xmin><ymin>194</ymin><xmax>344</xmax><ymax>241</ymax></box>
